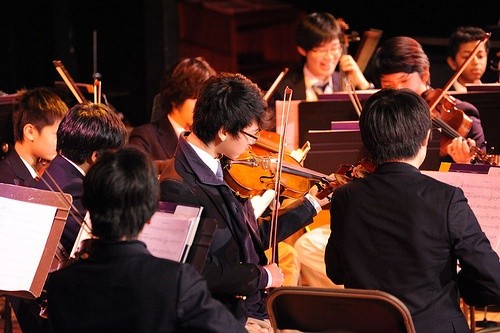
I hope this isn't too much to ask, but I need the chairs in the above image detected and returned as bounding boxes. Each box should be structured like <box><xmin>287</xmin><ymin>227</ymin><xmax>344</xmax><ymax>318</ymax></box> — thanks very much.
<box><xmin>265</xmin><ymin>286</ymin><xmax>416</xmax><ymax>333</ymax></box>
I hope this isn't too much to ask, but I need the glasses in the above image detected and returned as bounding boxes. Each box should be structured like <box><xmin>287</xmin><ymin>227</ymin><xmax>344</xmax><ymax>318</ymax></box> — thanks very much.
<box><xmin>240</xmin><ymin>130</ymin><xmax>260</xmax><ymax>144</ymax></box>
<box><xmin>311</xmin><ymin>46</ymin><xmax>343</xmax><ymax>56</ymax></box>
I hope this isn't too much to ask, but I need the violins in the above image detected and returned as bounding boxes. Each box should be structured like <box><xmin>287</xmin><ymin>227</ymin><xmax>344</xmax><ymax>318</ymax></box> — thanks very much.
<box><xmin>423</xmin><ymin>89</ymin><xmax>498</xmax><ymax>165</ymax></box>
<box><xmin>224</xmin><ymin>131</ymin><xmax>358</xmax><ymax>198</ymax></box>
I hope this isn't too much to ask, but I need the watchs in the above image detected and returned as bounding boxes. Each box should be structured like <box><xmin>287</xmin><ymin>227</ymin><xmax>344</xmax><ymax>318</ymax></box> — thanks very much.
<box><xmin>366</xmin><ymin>81</ymin><xmax>375</xmax><ymax>90</ymax></box>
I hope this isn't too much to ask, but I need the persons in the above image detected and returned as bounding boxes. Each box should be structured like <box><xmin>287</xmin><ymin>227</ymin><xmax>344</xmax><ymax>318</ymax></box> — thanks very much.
<box><xmin>41</xmin><ymin>144</ymin><xmax>247</xmax><ymax>333</ymax></box>
<box><xmin>0</xmin><ymin>87</ymin><xmax>69</xmax><ymax>188</ymax></box>
<box><xmin>34</xmin><ymin>101</ymin><xmax>129</xmax><ymax>258</ymax></box>
<box><xmin>274</xmin><ymin>10</ymin><xmax>375</xmax><ymax>102</ymax></box>
<box><xmin>158</xmin><ymin>71</ymin><xmax>338</xmax><ymax>319</ymax></box>
<box><xmin>372</xmin><ymin>35</ymin><xmax>486</xmax><ymax>166</ymax></box>
<box><xmin>444</xmin><ymin>26</ymin><xmax>491</xmax><ymax>92</ymax></box>
<box><xmin>128</xmin><ymin>55</ymin><xmax>219</xmax><ymax>174</ymax></box>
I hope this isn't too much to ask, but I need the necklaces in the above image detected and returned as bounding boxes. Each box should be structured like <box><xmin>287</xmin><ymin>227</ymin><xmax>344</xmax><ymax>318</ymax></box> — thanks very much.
<box><xmin>324</xmin><ymin>88</ymin><xmax>500</xmax><ymax>333</ymax></box>
